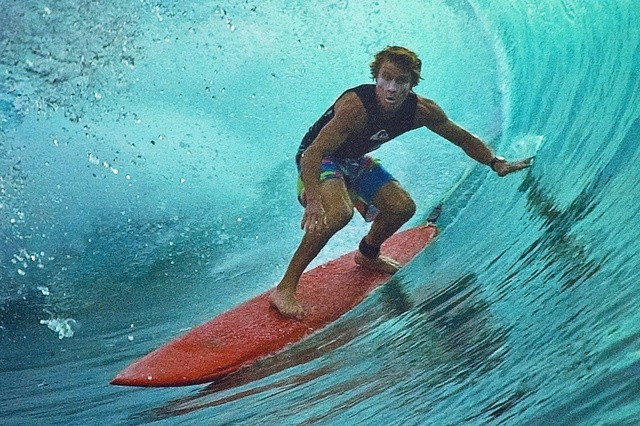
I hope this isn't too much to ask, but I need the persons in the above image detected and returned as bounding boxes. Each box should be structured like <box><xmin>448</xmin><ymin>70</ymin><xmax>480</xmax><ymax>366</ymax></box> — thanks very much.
<box><xmin>268</xmin><ymin>46</ymin><xmax>535</xmax><ymax>322</ymax></box>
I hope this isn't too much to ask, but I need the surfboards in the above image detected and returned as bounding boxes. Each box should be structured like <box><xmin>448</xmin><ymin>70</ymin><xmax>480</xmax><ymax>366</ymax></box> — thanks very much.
<box><xmin>110</xmin><ymin>224</ymin><xmax>437</xmax><ymax>388</ymax></box>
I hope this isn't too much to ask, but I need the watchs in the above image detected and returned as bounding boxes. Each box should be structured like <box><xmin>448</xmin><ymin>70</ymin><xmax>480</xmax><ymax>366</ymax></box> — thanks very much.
<box><xmin>490</xmin><ymin>156</ymin><xmax>508</xmax><ymax>172</ymax></box>
